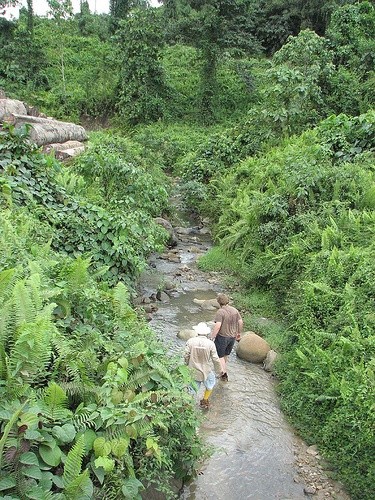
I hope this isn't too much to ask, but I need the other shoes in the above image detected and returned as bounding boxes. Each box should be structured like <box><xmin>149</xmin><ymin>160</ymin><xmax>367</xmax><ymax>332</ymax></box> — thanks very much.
<box><xmin>200</xmin><ymin>399</ymin><xmax>209</xmax><ymax>408</ymax></box>
<box><xmin>219</xmin><ymin>373</ymin><xmax>229</xmax><ymax>380</ymax></box>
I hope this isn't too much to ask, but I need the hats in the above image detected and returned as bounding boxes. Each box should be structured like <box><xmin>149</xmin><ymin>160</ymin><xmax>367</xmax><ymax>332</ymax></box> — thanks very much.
<box><xmin>192</xmin><ymin>322</ymin><xmax>211</xmax><ymax>334</ymax></box>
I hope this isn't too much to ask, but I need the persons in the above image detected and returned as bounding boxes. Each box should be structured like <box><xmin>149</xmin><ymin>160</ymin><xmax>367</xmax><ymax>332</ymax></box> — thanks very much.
<box><xmin>211</xmin><ymin>292</ymin><xmax>243</xmax><ymax>382</ymax></box>
<box><xmin>183</xmin><ymin>322</ymin><xmax>224</xmax><ymax>409</ymax></box>
